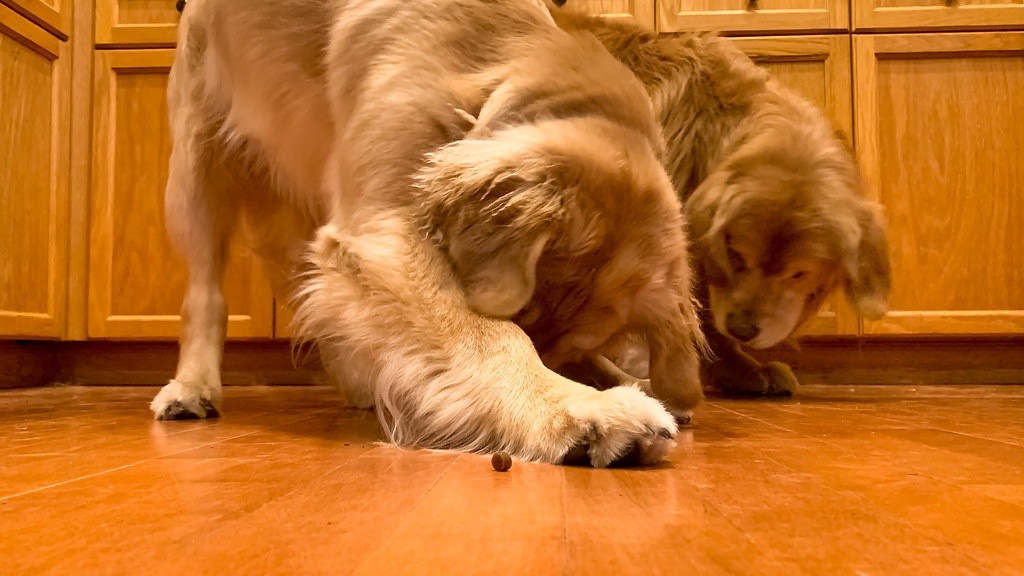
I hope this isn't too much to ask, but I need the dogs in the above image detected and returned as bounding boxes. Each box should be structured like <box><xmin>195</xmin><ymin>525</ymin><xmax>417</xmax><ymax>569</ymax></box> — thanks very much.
<box><xmin>149</xmin><ymin>0</ymin><xmax>892</xmax><ymax>467</ymax></box>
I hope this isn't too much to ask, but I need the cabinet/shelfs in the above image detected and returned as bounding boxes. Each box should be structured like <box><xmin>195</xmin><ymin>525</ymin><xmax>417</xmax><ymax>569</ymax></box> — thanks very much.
<box><xmin>0</xmin><ymin>0</ymin><xmax>74</xmax><ymax>392</ymax></box>
<box><xmin>654</xmin><ymin>0</ymin><xmax>1024</xmax><ymax>388</ymax></box>
<box><xmin>75</xmin><ymin>0</ymin><xmax>333</xmax><ymax>388</ymax></box>
